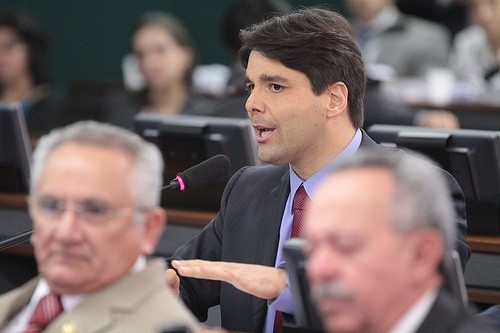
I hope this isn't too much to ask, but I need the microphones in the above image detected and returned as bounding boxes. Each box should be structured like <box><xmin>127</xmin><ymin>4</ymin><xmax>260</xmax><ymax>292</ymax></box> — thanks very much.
<box><xmin>170</xmin><ymin>154</ymin><xmax>230</xmax><ymax>191</ymax></box>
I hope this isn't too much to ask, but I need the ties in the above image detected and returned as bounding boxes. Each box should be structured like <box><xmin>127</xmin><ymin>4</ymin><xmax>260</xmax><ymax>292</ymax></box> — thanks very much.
<box><xmin>272</xmin><ymin>186</ymin><xmax>312</xmax><ymax>333</ymax></box>
<box><xmin>22</xmin><ymin>291</ymin><xmax>64</xmax><ymax>333</ymax></box>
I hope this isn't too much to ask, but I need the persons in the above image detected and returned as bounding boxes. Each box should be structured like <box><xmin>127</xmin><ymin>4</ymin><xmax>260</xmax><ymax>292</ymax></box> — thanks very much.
<box><xmin>0</xmin><ymin>120</ymin><xmax>197</xmax><ymax>333</ymax></box>
<box><xmin>107</xmin><ymin>11</ymin><xmax>230</xmax><ymax>133</ymax></box>
<box><xmin>0</xmin><ymin>9</ymin><xmax>72</xmax><ymax>140</ymax></box>
<box><xmin>344</xmin><ymin>0</ymin><xmax>449</xmax><ymax>77</ymax></box>
<box><xmin>162</xmin><ymin>8</ymin><xmax>471</xmax><ymax>333</ymax></box>
<box><xmin>451</xmin><ymin>0</ymin><xmax>500</xmax><ymax>102</ymax></box>
<box><xmin>301</xmin><ymin>150</ymin><xmax>500</xmax><ymax>333</ymax></box>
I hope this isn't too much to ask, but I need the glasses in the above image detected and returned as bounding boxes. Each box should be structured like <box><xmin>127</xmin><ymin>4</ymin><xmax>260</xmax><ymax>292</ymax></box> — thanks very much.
<box><xmin>28</xmin><ymin>193</ymin><xmax>133</xmax><ymax>225</ymax></box>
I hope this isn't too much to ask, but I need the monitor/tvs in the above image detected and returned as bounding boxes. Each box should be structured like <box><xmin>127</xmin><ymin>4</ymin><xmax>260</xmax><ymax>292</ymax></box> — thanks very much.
<box><xmin>134</xmin><ymin>114</ymin><xmax>261</xmax><ymax>212</ymax></box>
<box><xmin>368</xmin><ymin>124</ymin><xmax>500</xmax><ymax>236</ymax></box>
<box><xmin>0</xmin><ymin>100</ymin><xmax>34</xmax><ymax>193</ymax></box>
